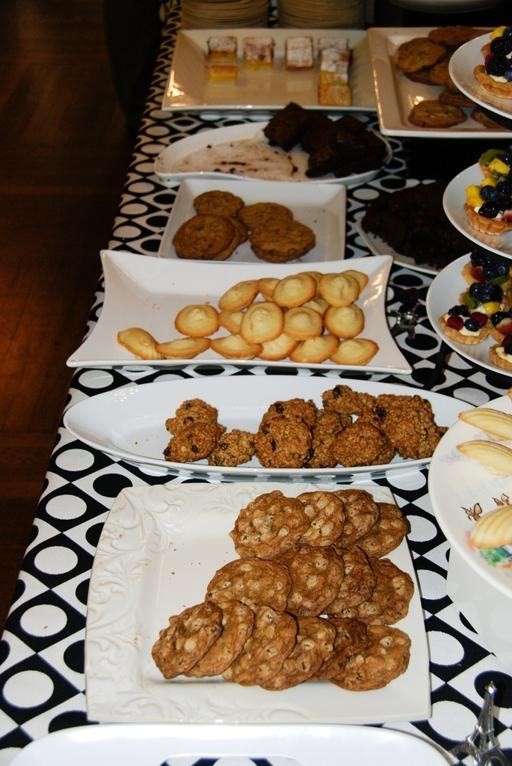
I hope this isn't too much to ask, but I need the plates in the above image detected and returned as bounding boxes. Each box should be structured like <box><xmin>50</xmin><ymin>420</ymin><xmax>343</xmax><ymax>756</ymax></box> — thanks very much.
<box><xmin>152</xmin><ymin>115</ymin><xmax>391</xmax><ymax>188</ymax></box>
<box><xmin>81</xmin><ymin>484</ymin><xmax>435</xmax><ymax>729</ymax></box>
<box><xmin>276</xmin><ymin>0</ymin><xmax>365</xmax><ymax>28</ymax></box>
<box><xmin>153</xmin><ymin>24</ymin><xmax>376</xmax><ymax>118</ymax></box>
<box><xmin>422</xmin><ymin>391</ymin><xmax>512</xmax><ymax>599</ymax></box>
<box><xmin>58</xmin><ymin>381</ymin><xmax>482</xmax><ymax>483</ymax></box>
<box><xmin>156</xmin><ymin>181</ymin><xmax>353</xmax><ymax>261</ymax></box>
<box><xmin>441</xmin><ymin>163</ymin><xmax>511</xmax><ymax>259</ymax></box>
<box><xmin>424</xmin><ymin>248</ymin><xmax>510</xmax><ymax>378</ymax></box>
<box><xmin>360</xmin><ymin>185</ymin><xmax>440</xmax><ymax>278</ymax></box>
<box><xmin>181</xmin><ymin>0</ymin><xmax>272</xmax><ymax>30</ymax></box>
<box><xmin>65</xmin><ymin>247</ymin><xmax>412</xmax><ymax>378</ymax></box>
<box><xmin>368</xmin><ymin>22</ymin><xmax>511</xmax><ymax>143</ymax></box>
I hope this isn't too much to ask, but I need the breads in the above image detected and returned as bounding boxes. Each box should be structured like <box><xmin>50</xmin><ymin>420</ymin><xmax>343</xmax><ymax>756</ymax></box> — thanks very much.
<box><xmin>456</xmin><ymin>408</ymin><xmax>512</xmax><ymax>547</ymax></box>
<box><xmin>118</xmin><ymin>270</ymin><xmax>380</xmax><ymax>366</ymax></box>
<box><xmin>172</xmin><ymin>189</ymin><xmax>316</xmax><ymax>262</ymax></box>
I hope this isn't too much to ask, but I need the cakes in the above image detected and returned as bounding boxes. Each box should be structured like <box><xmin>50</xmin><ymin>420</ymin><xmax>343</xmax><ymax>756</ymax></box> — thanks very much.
<box><xmin>464</xmin><ymin>150</ymin><xmax>512</xmax><ymax>236</ymax></box>
<box><xmin>438</xmin><ymin>247</ymin><xmax>512</xmax><ymax>372</ymax></box>
<box><xmin>206</xmin><ymin>35</ymin><xmax>388</xmax><ymax>177</ymax></box>
<box><xmin>474</xmin><ymin>26</ymin><xmax>512</xmax><ymax>101</ymax></box>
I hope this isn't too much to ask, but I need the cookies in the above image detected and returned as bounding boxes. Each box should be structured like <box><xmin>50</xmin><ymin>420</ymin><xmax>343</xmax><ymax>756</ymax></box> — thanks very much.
<box><xmin>394</xmin><ymin>26</ymin><xmax>508</xmax><ymax>132</ymax></box>
<box><xmin>152</xmin><ymin>489</ymin><xmax>413</xmax><ymax>691</ymax></box>
<box><xmin>163</xmin><ymin>384</ymin><xmax>449</xmax><ymax>469</ymax></box>
